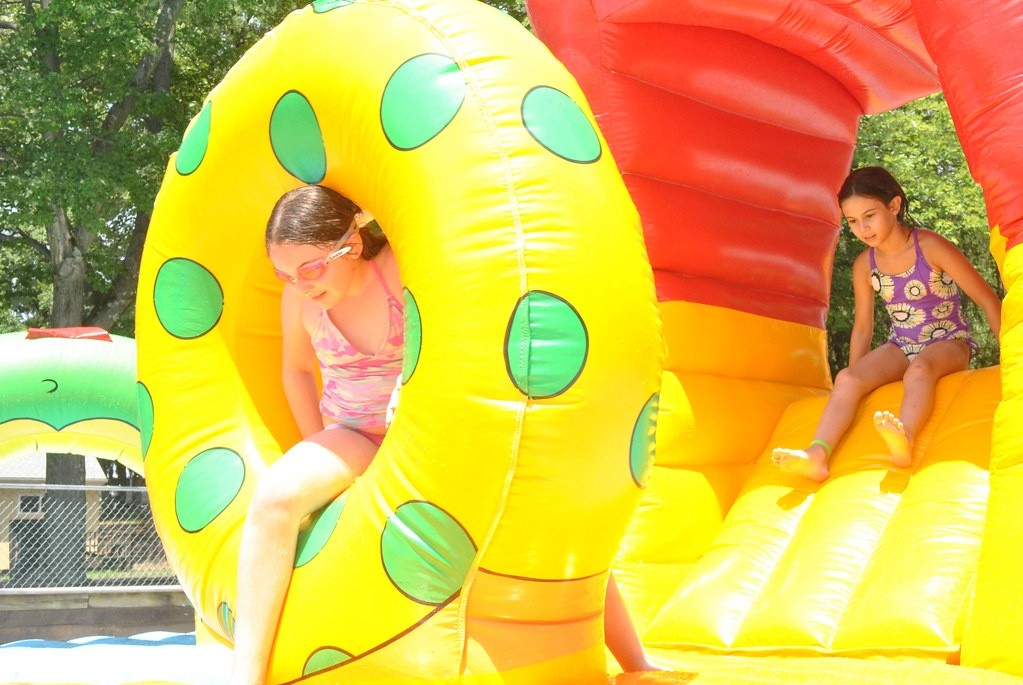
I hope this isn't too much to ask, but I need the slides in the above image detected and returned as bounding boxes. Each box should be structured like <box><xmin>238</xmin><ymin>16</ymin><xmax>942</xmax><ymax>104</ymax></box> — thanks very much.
<box><xmin>609</xmin><ymin>358</ymin><xmax>1023</xmax><ymax>685</ymax></box>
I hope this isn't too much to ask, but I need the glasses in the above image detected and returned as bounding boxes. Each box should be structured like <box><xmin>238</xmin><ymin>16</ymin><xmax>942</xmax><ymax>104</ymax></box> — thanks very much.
<box><xmin>274</xmin><ymin>246</ymin><xmax>352</xmax><ymax>282</ymax></box>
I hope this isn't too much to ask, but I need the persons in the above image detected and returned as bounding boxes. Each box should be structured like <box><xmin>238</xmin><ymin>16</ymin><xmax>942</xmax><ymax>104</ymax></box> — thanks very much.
<box><xmin>230</xmin><ymin>185</ymin><xmax>661</xmax><ymax>685</ymax></box>
<box><xmin>772</xmin><ymin>166</ymin><xmax>1001</xmax><ymax>482</ymax></box>
